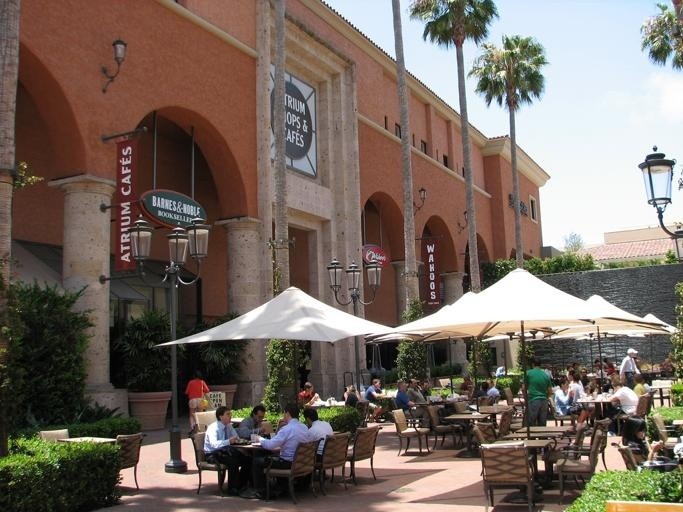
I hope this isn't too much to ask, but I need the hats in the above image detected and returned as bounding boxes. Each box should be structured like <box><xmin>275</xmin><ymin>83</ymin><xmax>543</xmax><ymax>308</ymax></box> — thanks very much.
<box><xmin>628</xmin><ymin>347</ymin><xmax>637</xmax><ymax>353</ymax></box>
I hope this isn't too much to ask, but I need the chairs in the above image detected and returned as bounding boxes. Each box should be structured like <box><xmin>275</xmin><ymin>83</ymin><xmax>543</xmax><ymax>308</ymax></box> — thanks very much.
<box><xmin>115</xmin><ymin>431</ymin><xmax>144</xmax><ymax>489</ymax></box>
<box><xmin>186</xmin><ymin>369</ymin><xmax>682</xmax><ymax>512</ymax></box>
<box><xmin>37</xmin><ymin>428</ymin><xmax>68</xmax><ymax>445</ymax></box>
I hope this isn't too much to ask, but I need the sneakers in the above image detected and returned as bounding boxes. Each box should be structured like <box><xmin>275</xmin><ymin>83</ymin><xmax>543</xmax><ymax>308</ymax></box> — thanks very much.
<box><xmin>239</xmin><ymin>489</ymin><xmax>257</xmax><ymax>498</ymax></box>
<box><xmin>227</xmin><ymin>486</ymin><xmax>239</xmax><ymax>495</ymax></box>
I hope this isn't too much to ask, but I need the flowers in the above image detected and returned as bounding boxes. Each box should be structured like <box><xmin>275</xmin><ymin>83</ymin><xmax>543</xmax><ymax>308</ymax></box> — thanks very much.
<box><xmin>10</xmin><ymin>158</ymin><xmax>43</xmax><ymax>204</ymax></box>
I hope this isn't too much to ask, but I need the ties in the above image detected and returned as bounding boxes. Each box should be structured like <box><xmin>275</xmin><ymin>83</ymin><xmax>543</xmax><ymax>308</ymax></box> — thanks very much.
<box><xmin>225</xmin><ymin>427</ymin><xmax>228</xmax><ymax>440</ymax></box>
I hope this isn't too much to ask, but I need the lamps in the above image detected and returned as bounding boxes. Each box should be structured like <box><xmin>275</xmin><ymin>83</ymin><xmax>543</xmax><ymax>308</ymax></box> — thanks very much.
<box><xmin>102</xmin><ymin>38</ymin><xmax>126</xmax><ymax>92</ymax></box>
<box><xmin>457</xmin><ymin>210</ymin><xmax>468</xmax><ymax>234</ymax></box>
<box><xmin>414</xmin><ymin>185</ymin><xmax>427</xmax><ymax>217</ymax></box>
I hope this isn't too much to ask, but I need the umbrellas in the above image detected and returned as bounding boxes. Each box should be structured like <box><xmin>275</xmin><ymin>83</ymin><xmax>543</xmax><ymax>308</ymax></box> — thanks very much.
<box><xmin>150</xmin><ymin>285</ymin><xmax>394</xmax><ymax>412</ymax></box>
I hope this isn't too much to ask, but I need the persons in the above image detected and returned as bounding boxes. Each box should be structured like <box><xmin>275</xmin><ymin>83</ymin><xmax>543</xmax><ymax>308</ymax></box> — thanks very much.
<box><xmin>461</xmin><ymin>376</ymin><xmax>499</xmax><ymax>401</ymax></box>
<box><xmin>204</xmin><ymin>403</ymin><xmax>333</xmax><ymax>498</ymax></box>
<box><xmin>344</xmin><ymin>378</ymin><xmax>382</xmax><ymax>407</ymax></box>
<box><xmin>184</xmin><ymin>370</ymin><xmax>211</xmax><ymax>435</ymax></box>
<box><xmin>299</xmin><ymin>382</ymin><xmax>318</xmax><ymax>407</ymax></box>
<box><xmin>521</xmin><ymin>357</ymin><xmax>552</xmax><ymax>427</ymax></box>
<box><xmin>395</xmin><ymin>378</ymin><xmax>431</xmax><ymax>427</ymax></box>
<box><xmin>551</xmin><ymin>348</ymin><xmax>675</xmax><ymax>430</ymax></box>
<box><xmin>177</xmin><ymin>369</ymin><xmax>187</xmax><ymax>417</ymax></box>
<box><xmin>621</xmin><ymin>415</ymin><xmax>671</xmax><ymax>471</ymax></box>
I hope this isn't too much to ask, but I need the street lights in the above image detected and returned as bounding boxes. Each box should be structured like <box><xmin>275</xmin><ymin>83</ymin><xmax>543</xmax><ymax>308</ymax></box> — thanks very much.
<box><xmin>125</xmin><ymin>209</ymin><xmax>212</xmax><ymax>475</ymax></box>
<box><xmin>634</xmin><ymin>143</ymin><xmax>683</xmax><ymax>239</ymax></box>
<box><xmin>326</xmin><ymin>255</ymin><xmax>383</xmax><ymax>391</ymax></box>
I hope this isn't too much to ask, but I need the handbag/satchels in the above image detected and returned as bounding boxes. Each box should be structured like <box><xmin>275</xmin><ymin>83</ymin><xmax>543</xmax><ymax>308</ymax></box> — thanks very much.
<box><xmin>200</xmin><ymin>400</ymin><xmax>208</xmax><ymax>409</ymax></box>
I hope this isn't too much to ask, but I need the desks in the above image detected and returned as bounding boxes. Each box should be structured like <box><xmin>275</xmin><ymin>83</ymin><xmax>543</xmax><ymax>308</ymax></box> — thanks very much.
<box><xmin>55</xmin><ymin>436</ymin><xmax>117</xmax><ymax>445</ymax></box>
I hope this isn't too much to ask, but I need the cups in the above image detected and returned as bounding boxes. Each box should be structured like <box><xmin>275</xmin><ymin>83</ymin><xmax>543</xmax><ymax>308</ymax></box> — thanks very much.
<box><xmin>571</xmin><ymin>386</ymin><xmax>617</xmax><ymax>403</ymax></box>
<box><xmin>229</xmin><ymin>421</ymin><xmax>277</xmax><ymax>449</ymax></box>
